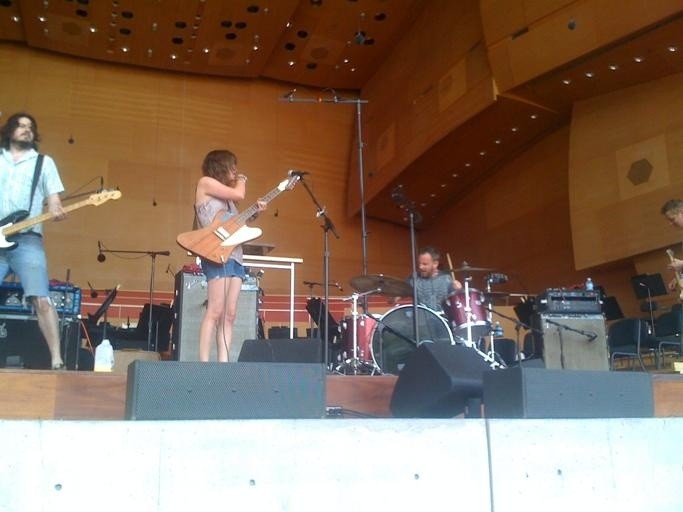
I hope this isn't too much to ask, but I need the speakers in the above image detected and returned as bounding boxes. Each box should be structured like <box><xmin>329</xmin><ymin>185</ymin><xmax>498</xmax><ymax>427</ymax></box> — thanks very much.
<box><xmin>0</xmin><ymin>311</ymin><xmax>82</xmax><ymax>371</ymax></box>
<box><xmin>539</xmin><ymin>313</ymin><xmax>609</xmax><ymax>371</ymax></box>
<box><xmin>482</xmin><ymin>367</ymin><xmax>656</xmax><ymax>418</ymax></box>
<box><xmin>172</xmin><ymin>270</ymin><xmax>259</xmax><ymax>363</ymax></box>
<box><xmin>124</xmin><ymin>358</ymin><xmax>326</xmax><ymax>420</ymax></box>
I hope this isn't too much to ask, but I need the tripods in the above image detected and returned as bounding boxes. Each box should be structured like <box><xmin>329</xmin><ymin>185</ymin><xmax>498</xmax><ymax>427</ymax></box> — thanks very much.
<box><xmin>329</xmin><ymin>292</ymin><xmax>385</xmax><ymax>376</ymax></box>
<box><xmin>484</xmin><ymin>279</ymin><xmax>508</xmax><ymax>371</ymax></box>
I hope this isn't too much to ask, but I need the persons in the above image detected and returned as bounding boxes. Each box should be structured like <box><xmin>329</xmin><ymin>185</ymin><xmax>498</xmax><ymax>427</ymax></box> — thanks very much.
<box><xmin>0</xmin><ymin>113</ymin><xmax>68</xmax><ymax>371</ymax></box>
<box><xmin>394</xmin><ymin>246</ymin><xmax>462</xmax><ymax>315</ymax></box>
<box><xmin>195</xmin><ymin>150</ymin><xmax>267</xmax><ymax>364</ymax></box>
<box><xmin>661</xmin><ymin>200</ymin><xmax>683</xmax><ymax>290</ymax></box>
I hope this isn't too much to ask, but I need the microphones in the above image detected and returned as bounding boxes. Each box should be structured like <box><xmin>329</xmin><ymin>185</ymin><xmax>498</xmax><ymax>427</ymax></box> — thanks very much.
<box><xmin>588</xmin><ymin>335</ymin><xmax>598</xmax><ymax>342</ymax></box>
<box><xmin>283</xmin><ymin>88</ymin><xmax>297</xmax><ymax>99</ymax></box>
<box><xmin>97</xmin><ymin>240</ymin><xmax>106</xmax><ymax>263</ymax></box>
<box><xmin>334</xmin><ymin>280</ymin><xmax>343</xmax><ymax>291</ymax></box>
<box><xmin>288</xmin><ymin>169</ymin><xmax>310</xmax><ymax>177</ymax></box>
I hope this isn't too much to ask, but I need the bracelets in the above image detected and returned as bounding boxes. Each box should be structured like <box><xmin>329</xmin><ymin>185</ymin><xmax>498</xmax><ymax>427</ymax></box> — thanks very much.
<box><xmin>238</xmin><ymin>175</ymin><xmax>247</xmax><ymax>182</ymax></box>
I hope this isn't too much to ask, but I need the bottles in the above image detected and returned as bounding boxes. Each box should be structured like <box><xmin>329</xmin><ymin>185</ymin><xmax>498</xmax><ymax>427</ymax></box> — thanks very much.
<box><xmin>93</xmin><ymin>339</ymin><xmax>113</xmax><ymax>373</ymax></box>
<box><xmin>495</xmin><ymin>321</ymin><xmax>504</xmax><ymax>337</ymax></box>
<box><xmin>585</xmin><ymin>277</ymin><xmax>594</xmax><ymax>291</ymax></box>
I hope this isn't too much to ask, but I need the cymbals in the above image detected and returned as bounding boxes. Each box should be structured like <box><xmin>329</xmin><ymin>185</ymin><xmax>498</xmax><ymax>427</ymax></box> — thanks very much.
<box><xmin>351</xmin><ymin>274</ymin><xmax>414</xmax><ymax>298</ymax></box>
<box><xmin>440</xmin><ymin>265</ymin><xmax>498</xmax><ymax>274</ymax></box>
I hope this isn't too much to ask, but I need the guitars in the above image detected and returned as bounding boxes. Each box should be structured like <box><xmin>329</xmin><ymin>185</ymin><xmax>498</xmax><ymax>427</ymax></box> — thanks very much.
<box><xmin>177</xmin><ymin>174</ymin><xmax>300</xmax><ymax>264</ymax></box>
<box><xmin>666</xmin><ymin>248</ymin><xmax>683</xmax><ymax>299</ymax></box>
<box><xmin>0</xmin><ymin>189</ymin><xmax>121</xmax><ymax>253</ymax></box>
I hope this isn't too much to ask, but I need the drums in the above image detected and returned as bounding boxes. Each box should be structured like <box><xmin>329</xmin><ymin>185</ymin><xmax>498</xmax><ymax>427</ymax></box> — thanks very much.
<box><xmin>370</xmin><ymin>302</ymin><xmax>454</xmax><ymax>376</ymax></box>
<box><xmin>443</xmin><ymin>287</ymin><xmax>491</xmax><ymax>336</ymax></box>
<box><xmin>334</xmin><ymin>312</ymin><xmax>382</xmax><ymax>376</ymax></box>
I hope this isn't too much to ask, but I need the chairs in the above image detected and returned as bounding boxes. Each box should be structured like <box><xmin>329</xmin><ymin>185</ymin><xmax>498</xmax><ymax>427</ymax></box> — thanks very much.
<box><xmin>622</xmin><ymin>304</ymin><xmax>683</xmax><ymax>372</ymax></box>
<box><xmin>608</xmin><ymin>318</ymin><xmax>647</xmax><ymax>372</ymax></box>
